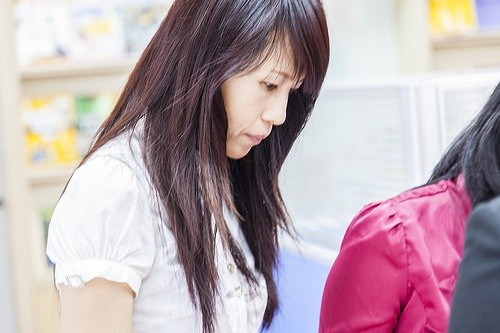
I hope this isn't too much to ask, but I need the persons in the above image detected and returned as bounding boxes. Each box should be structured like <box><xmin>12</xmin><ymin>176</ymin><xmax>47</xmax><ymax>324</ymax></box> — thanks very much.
<box><xmin>45</xmin><ymin>1</ymin><xmax>330</xmax><ymax>331</ymax></box>
<box><xmin>318</xmin><ymin>79</ymin><xmax>500</xmax><ymax>333</ymax></box>
<box><xmin>444</xmin><ymin>105</ymin><xmax>499</xmax><ymax>332</ymax></box>
